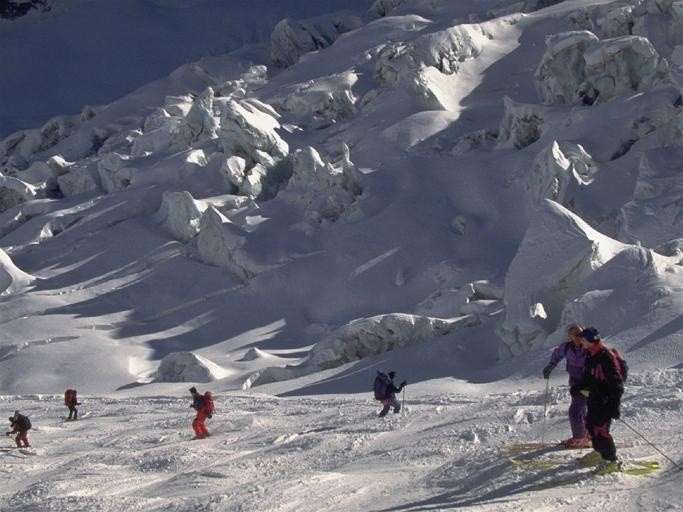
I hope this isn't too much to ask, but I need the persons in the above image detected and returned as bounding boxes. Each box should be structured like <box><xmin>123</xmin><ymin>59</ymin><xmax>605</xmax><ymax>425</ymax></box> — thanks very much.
<box><xmin>573</xmin><ymin>328</ymin><xmax>626</xmax><ymax>475</ymax></box>
<box><xmin>62</xmin><ymin>389</ymin><xmax>81</xmax><ymax>420</ymax></box>
<box><xmin>5</xmin><ymin>409</ymin><xmax>31</xmax><ymax>448</ymax></box>
<box><xmin>188</xmin><ymin>386</ymin><xmax>213</xmax><ymax>440</ymax></box>
<box><xmin>373</xmin><ymin>371</ymin><xmax>407</xmax><ymax>417</ymax></box>
<box><xmin>543</xmin><ymin>322</ymin><xmax>592</xmax><ymax>448</ymax></box>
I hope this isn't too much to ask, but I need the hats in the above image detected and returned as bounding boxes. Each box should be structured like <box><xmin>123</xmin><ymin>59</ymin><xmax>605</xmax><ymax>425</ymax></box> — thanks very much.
<box><xmin>388</xmin><ymin>371</ymin><xmax>395</xmax><ymax>376</ymax></box>
<box><xmin>565</xmin><ymin>322</ymin><xmax>584</xmax><ymax>340</ymax></box>
<box><xmin>576</xmin><ymin>327</ymin><xmax>600</xmax><ymax>341</ymax></box>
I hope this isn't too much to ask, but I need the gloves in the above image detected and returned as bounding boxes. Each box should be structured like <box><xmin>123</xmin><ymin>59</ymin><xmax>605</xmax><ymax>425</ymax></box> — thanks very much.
<box><xmin>570</xmin><ymin>384</ymin><xmax>584</xmax><ymax>396</ymax></box>
<box><xmin>401</xmin><ymin>381</ymin><xmax>407</xmax><ymax>387</ymax></box>
<box><xmin>543</xmin><ymin>363</ymin><xmax>556</xmax><ymax>379</ymax></box>
<box><xmin>604</xmin><ymin>398</ymin><xmax>622</xmax><ymax>420</ymax></box>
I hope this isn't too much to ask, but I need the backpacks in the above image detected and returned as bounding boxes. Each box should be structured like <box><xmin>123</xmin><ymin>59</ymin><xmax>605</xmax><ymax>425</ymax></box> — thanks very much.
<box><xmin>202</xmin><ymin>390</ymin><xmax>214</xmax><ymax>418</ymax></box>
<box><xmin>374</xmin><ymin>369</ymin><xmax>390</xmax><ymax>400</ymax></box>
<box><xmin>17</xmin><ymin>413</ymin><xmax>32</xmax><ymax>431</ymax></box>
<box><xmin>602</xmin><ymin>345</ymin><xmax>628</xmax><ymax>383</ymax></box>
<box><xmin>62</xmin><ymin>388</ymin><xmax>77</xmax><ymax>406</ymax></box>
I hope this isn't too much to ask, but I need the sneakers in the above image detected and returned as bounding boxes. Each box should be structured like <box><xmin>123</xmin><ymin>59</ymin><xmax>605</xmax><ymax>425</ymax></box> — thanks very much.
<box><xmin>562</xmin><ymin>437</ymin><xmax>590</xmax><ymax>449</ymax></box>
<box><xmin>575</xmin><ymin>450</ymin><xmax>603</xmax><ymax>464</ymax></box>
<box><xmin>592</xmin><ymin>461</ymin><xmax>618</xmax><ymax>475</ymax></box>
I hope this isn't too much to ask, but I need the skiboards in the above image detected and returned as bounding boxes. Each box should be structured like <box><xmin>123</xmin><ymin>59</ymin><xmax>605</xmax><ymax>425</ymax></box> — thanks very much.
<box><xmin>510</xmin><ymin>441</ymin><xmax>633</xmax><ymax>452</ymax></box>
<box><xmin>508</xmin><ymin>457</ymin><xmax>660</xmax><ymax>491</ymax></box>
